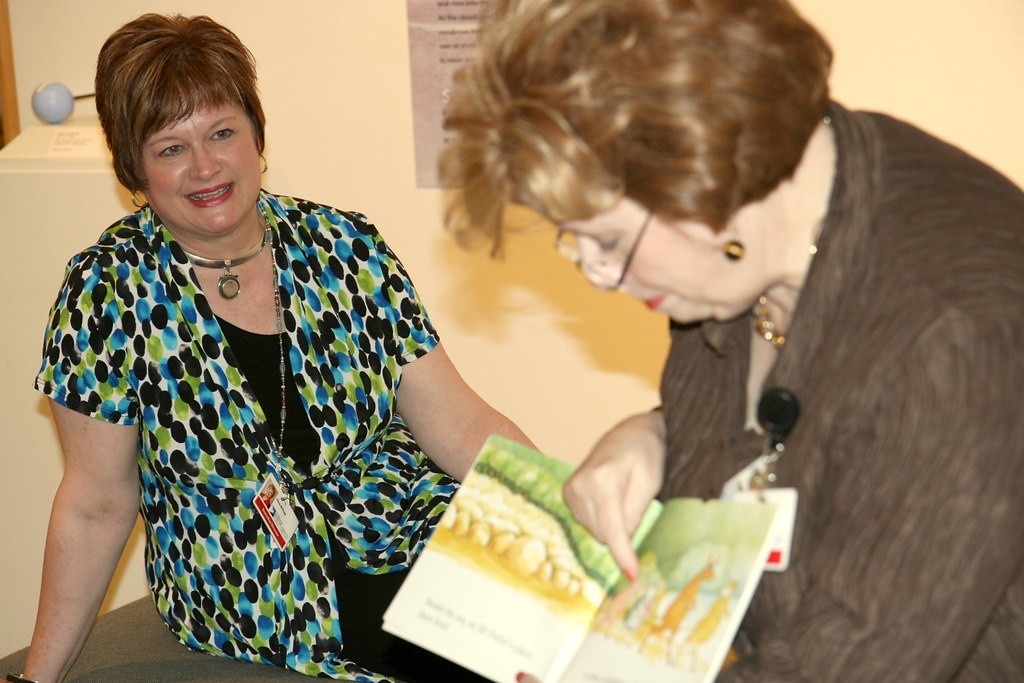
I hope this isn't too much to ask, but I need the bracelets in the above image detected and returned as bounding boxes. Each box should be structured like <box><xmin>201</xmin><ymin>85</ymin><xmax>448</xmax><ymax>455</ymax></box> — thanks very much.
<box><xmin>6</xmin><ymin>673</ymin><xmax>39</xmax><ymax>683</ymax></box>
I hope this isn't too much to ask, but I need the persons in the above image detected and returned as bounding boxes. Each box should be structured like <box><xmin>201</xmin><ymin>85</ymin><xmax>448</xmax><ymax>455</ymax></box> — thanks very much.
<box><xmin>436</xmin><ymin>1</ymin><xmax>1023</xmax><ymax>683</ymax></box>
<box><xmin>0</xmin><ymin>11</ymin><xmax>549</xmax><ymax>683</ymax></box>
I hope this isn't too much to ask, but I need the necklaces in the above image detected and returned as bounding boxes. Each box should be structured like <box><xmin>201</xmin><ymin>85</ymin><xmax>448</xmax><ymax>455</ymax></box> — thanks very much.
<box><xmin>179</xmin><ymin>227</ymin><xmax>269</xmax><ymax>300</ymax></box>
<box><xmin>158</xmin><ymin>197</ymin><xmax>286</xmax><ymax>478</ymax></box>
<box><xmin>750</xmin><ymin>292</ymin><xmax>789</xmax><ymax>352</ymax></box>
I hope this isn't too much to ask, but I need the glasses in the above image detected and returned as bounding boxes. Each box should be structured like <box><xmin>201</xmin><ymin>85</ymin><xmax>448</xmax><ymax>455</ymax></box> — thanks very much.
<box><xmin>555</xmin><ymin>191</ymin><xmax>656</xmax><ymax>293</ymax></box>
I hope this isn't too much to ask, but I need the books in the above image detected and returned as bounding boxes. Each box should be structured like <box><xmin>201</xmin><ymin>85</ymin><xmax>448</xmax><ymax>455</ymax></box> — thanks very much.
<box><xmin>380</xmin><ymin>431</ymin><xmax>785</xmax><ymax>683</ymax></box>
